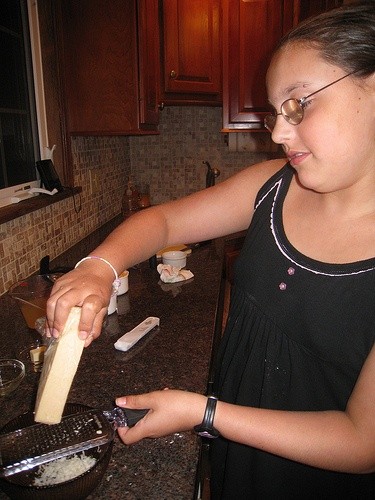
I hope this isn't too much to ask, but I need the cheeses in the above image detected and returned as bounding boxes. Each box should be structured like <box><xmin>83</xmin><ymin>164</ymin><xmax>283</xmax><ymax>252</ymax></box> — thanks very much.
<box><xmin>33</xmin><ymin>307</ymin><xmax>87</xmax><ymax>423</ymax></box>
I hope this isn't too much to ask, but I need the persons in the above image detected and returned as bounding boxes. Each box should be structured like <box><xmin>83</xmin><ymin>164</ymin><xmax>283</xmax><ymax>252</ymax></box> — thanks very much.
<box><xmin>46</xmin><ymin>7</ymin><xmax>375</xmax><ymax>500</ymax></box>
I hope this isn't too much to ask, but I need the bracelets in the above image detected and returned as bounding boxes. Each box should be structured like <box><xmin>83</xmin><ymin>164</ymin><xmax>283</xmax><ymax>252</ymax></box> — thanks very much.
<box><xmin>74</xmin><ymin>255</ymin><xmax>121</xmax><ymax>298</ymax></box>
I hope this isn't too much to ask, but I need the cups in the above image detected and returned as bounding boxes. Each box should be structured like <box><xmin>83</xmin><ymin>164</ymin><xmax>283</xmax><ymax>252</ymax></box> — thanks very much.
<box><xmin>7</xmin><ymin>274</ymin><xmax>60</xmax><ymax>329</ymax></box>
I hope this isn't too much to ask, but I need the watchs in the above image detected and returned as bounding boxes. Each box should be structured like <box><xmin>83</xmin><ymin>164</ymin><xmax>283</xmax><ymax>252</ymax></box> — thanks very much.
<box><xmin>193</xmin><ymin>395</ymin><xmax>219</xmax><ymax>438</ymax></box>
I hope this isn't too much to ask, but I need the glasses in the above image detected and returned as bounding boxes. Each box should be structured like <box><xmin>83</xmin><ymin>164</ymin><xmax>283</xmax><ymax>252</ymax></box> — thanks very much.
<box><xmin>263</xmin><ymin>67</ymin><xmax>368</xmax><ymax>134</ymax></box>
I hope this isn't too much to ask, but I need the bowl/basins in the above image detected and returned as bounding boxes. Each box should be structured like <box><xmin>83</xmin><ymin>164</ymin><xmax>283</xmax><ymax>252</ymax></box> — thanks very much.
<box><xmin>117</xmin><ymin>270</ymin><xmax>131</xmax><ymax>295</ymax></box>
<box><xmin>0</xmin><ymin>358</ymin><xmax>25</xmax><ymax>401</ymax></box>
<box><xmin>15</xmin><ymin>338</ymin><xmax>48</xmax><ymax>373</ymax></box>
<box><xmin>0</xmin><ymin>401</ymin><xmax>115</xmax><ymax>499</ymax></box>
<box><xmin>162</xmin><ymin>250</ymin><xmax>187</xmax><ymax>269</ymax></box>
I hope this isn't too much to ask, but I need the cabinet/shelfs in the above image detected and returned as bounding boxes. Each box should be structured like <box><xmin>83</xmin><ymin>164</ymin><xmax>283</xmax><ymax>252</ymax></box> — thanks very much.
<box><xmin>54</xmin><ymin>0</ymin><xmax>303</xmax><ymax>134</ymax></box>
